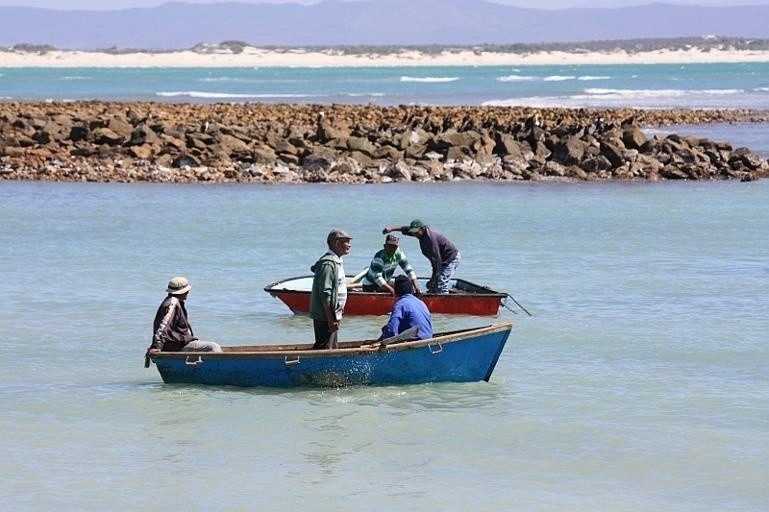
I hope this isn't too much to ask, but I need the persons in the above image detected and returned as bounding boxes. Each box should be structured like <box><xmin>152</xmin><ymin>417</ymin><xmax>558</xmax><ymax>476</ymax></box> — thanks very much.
<box><xmin>382</xmin><ymin>218</ymin><xmax>462</xmax><ymax>295</ymax></box>
<box><xmin>148</xmin><ymin>277</ymin><xmax>224</xmax><ymax>354</ymax></box>
<box><xmin>363</xmin><ymin>234</ymin><xmax>423</xmax><ymax>298</ymax></box>
<box><xmin>376</xmin><ymin>274</ymin><xmax>433</xmax><ymax>342</ymax></box>
<box><xmin>310</xmin><ymin>228</ymin><xmax>352</xmax><ymax>349</ymax></box>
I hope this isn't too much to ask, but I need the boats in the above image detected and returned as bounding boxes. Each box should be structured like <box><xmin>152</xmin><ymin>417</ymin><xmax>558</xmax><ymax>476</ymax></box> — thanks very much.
<box><xmin>145</xmin><ymin>322</ymin><xmax>513</xmax><ymax>390</ymax></box>
<box><xmin>262</xmin><ymin>276</ymin><xmax>510</xmax><ymax>320</ymax></box>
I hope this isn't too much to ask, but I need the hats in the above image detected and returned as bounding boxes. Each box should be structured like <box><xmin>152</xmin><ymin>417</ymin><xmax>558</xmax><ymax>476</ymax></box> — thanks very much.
<box><xmin>408</xmin><ymin>219</ymin><xmax>424</xmax><ymax>233</ymax></box>
<box><xmin>166</xmin><ymin>277</ymin><xmax>192</xmax><ymax>294</ymax></box>
<box><xmin>395</xmin><ymin>275</ymin><xmax>412</xmax><ymax>295</ymax></box>
<box><xmin>386</xmin><ymin>234</ymin><xmax>399</xmax><ymax>247</ymax></box>
<box><xmin>327</xmin><ymin>228</ymin><xmax>352</xmax><ymax>242</ymax></box>
